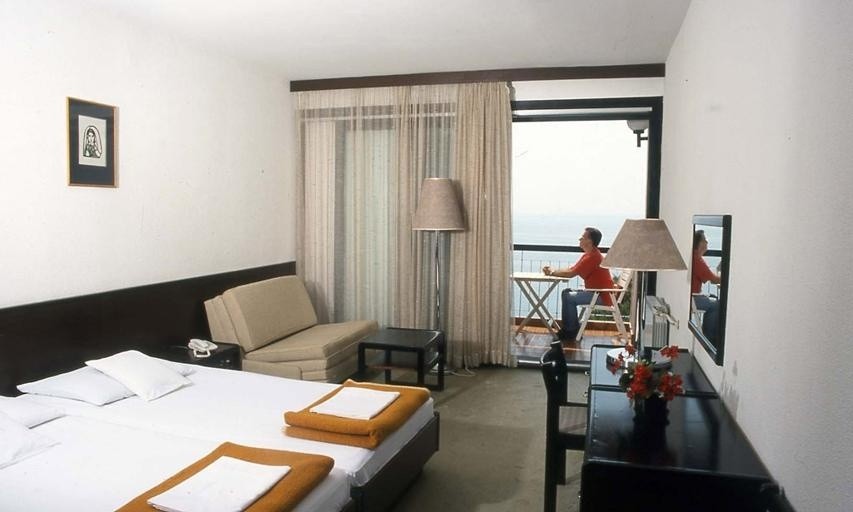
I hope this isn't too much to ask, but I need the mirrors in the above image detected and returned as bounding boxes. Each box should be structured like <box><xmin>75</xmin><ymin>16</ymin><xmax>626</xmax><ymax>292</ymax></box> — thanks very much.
<box><xmin>687</xmin><ymin>215</ymin><xmax>732</xmax><ymax>366</ymax></box>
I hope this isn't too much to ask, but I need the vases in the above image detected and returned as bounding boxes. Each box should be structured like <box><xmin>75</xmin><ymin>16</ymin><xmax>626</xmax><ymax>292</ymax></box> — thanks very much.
<box><xmin>632</xmin><ymin>393</ymin><xmax>670</xmax><ymax>447</ymax></box>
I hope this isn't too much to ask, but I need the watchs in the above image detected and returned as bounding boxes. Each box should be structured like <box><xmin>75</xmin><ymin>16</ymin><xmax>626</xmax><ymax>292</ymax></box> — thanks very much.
<box><xmin>550</xmin><ymin>271</ymin><xmax>553</xmax><ymax>276</ymax></box>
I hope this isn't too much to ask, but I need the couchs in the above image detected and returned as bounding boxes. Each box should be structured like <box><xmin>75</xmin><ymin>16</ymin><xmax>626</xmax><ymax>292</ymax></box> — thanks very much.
<box><xmin>204</xmin><ymin>275</ymin><xmax>380</xmax><ymax>383</ymax></box>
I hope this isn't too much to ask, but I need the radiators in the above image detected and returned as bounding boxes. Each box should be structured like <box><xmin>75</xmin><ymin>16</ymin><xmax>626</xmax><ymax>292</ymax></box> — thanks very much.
<box><xmin>642</xmin><ymin>295</ymin><xmax>679</xmax><ymax>348</ymax></box>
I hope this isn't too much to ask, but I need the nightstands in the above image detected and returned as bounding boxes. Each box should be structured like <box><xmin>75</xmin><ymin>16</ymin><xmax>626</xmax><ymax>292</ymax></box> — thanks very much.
<box><xmin>161</xmin><ymin>339</ymin><xmax>242</xmax><ymax>370</ymax></box>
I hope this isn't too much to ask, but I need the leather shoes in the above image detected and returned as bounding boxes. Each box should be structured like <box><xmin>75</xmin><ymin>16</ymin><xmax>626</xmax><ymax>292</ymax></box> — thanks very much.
<box><xmin>551</xmin><ymin>340</ymin><xmax>561</xmax><ymax>346</ymax></box>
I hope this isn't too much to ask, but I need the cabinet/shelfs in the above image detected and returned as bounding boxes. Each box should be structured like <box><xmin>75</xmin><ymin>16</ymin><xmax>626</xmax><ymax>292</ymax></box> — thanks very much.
<box><xmin>585</xmin><ymin>343</ymin><xmax>716</xmax><ymax>397</ymax></box>
<box><xmin>579</xmin><ymin>389</ymin><xmax>772</xmax><ymax>511</ymax></box>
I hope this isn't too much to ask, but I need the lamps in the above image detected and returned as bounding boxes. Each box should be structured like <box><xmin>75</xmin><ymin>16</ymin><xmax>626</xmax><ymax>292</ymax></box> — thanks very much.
<box><xmin>626</xmin><ymin>119</ymin><xmax>648</xmax><ymax>146</ymax></box>
<box><xmin>410</xmin><ymin>178</ymin><xmax>465</xmax><ymax>374</ymax></box>
<box><xmin>600</xmin><ymin>219</ymin><xmax>688</xmax><ymax>371</ymax></box>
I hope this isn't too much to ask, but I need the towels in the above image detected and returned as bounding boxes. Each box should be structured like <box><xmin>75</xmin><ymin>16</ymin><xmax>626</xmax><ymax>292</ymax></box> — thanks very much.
<box><xmin>147</xmin><ymin>455</ymin><xmax>291</xmax><ymax>512</ymax></box>
<box><xmin>309</xmin><ymin>387</ymin><xmax>400</xmax><ymax>420</ymax></box>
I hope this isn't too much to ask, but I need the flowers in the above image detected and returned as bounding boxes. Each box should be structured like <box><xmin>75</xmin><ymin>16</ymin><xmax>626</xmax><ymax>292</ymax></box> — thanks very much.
<box><xmin>611</xmin><ymin>340</ymin><xmax>685</xmax><ymax>401</ymax></box>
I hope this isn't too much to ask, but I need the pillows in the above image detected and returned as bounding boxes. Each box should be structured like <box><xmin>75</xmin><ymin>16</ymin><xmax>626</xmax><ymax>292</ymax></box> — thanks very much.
<box><xmin>0</xmin><ymin>396</ymin><xmax>65</xmax><ymax>429</ymax></box>
<box><xmin>16</xmin><ymin>356</ymin><xmax>194</xmax><ymax>405</ymax></box>
<box><xmin>85</xmin><ymin>351</ymin><xmax>191</xmax><ymax>401</ymax></box>
<box><xmin>0</xmin><ymin>411</ymin><xmax>58</xmax><ymax>469</ymax></box>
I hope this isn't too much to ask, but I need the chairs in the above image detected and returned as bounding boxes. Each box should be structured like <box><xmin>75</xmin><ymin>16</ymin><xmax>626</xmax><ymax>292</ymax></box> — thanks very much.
<box><xmin>576</xmin><ymin>270</ymin><xmax>635</xmax><ymax>341</ymax></box>
<box><xmin>540</xmin><ymin>340</ymin><xmax>590</xmax><ymax>512</ymax></box>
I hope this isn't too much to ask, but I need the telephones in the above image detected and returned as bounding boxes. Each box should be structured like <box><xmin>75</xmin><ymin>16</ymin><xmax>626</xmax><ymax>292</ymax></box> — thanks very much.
<box><xmin>188</xmin><ymin>338</ymin><xmax>218</xmax><ymax>358</ymax></box>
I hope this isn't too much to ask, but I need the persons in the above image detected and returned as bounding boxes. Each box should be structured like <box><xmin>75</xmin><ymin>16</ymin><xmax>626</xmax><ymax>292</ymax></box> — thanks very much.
<box><xmin>690</xmin><ymin>229</ymin><xmax>721</xmax><ymax>348</ymax></box>
<box><xmin>83</xmin><ymin>125</ymin><xmax>103</xmax><ymax>158</ymax></box>
<box><xmin>543</xmin><ymin>227</ymin><xmax>615</xmax><ymax>342</ymax></box>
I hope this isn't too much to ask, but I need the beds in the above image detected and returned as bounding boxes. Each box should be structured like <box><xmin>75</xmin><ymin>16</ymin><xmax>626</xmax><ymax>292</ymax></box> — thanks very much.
<box><xmin>14</xmin><ymin>365</ymin><xmax>440</xmax><ymax>512</ymax></box>
<box><xmin>1</xmin><ymin>414</ymin><xmax>355</xmax><ymax>512</ymax></box>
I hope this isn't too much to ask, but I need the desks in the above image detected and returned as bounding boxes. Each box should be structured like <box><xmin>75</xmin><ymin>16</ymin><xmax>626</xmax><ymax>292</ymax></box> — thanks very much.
<box><xmin>510</xmin><ymin>273</ymin><xmax>574</xmax><ymax>341</ymax></box>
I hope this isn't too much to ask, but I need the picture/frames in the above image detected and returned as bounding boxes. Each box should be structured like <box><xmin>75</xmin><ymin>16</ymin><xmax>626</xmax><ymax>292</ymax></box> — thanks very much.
<box><xmin>65</xmin><ymin>97</ymin><xmax>120</xmax><ymax>187</ymax></box>
